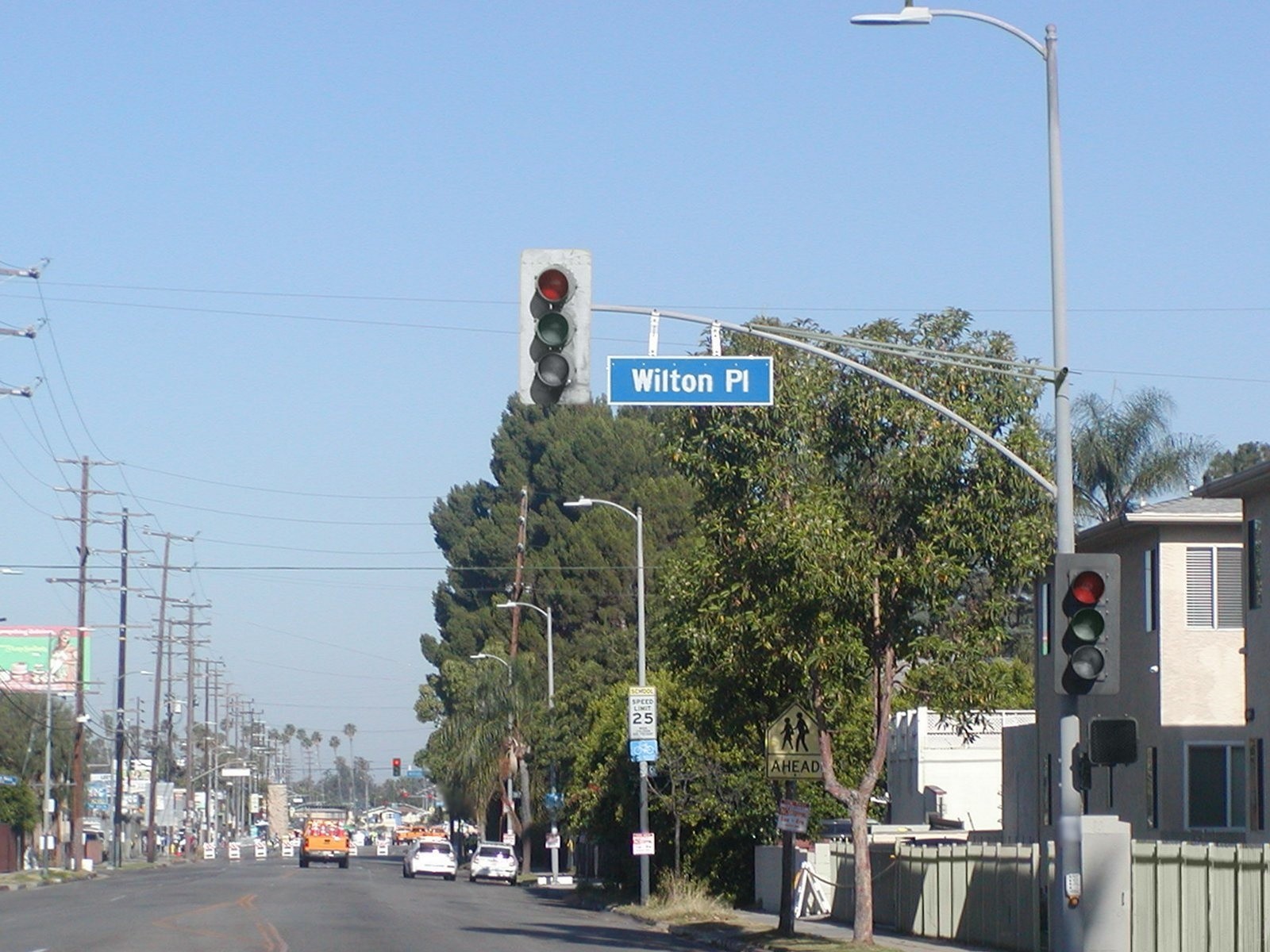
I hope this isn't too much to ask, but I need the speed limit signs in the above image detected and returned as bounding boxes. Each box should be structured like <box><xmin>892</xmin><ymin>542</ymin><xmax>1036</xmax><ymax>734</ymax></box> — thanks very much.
<box><xmin>628</xmin><ymin>696</ymin><xmax>657</xmax><ymax>740</ymax></box>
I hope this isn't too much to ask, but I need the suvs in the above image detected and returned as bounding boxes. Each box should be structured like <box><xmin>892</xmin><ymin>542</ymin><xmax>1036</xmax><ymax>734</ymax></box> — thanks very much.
<box><xmin>297</xmin><ymin>813</ymin><xmax>352</xmax><ymax>870</ymax></box>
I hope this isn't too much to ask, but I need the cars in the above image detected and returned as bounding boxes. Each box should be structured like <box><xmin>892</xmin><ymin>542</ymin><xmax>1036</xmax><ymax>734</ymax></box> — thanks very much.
<box><xmin>402</xmin><ymin>836</ymin><xmax>459</xmax><ymax>881</ymax></box>
<box><xmin>255</xmin><ymin>818</ymin><xmax>478</xmax><ymax>850</ymax></box>
<box><xmin>468</xmin><ymin>842</ymin><xmax>521</xmax><ymax>886</ymax></box>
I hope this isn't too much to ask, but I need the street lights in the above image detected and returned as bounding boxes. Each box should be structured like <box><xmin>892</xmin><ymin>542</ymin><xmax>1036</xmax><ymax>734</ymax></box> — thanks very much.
<box><xmin>847</xmin><ymin>0</ymin><xmax>1087</xmax><ymax>951</ymax></box>
<box><xmin>43</xmin><ymin>626</ymin><xmax>90</xmax><ymax>873</ymax></box>
<box><xmin>470</xmin><ymin>653</ymin><xmax>514</xmax><ymax>832</ymax></box>
<box><xmin>565</xmin><ymin>495</ymin><xmax>650</xmax><ymax>909</ymax></box>
<box><xmin>495</xmin><ymin>599</ymin><xmax>559</xmax><ymax>885</ymax></box>
<box><xmin>109</xmin><ymin>670</ymin><xmax>156</xmax><ymax>867</ymax></box>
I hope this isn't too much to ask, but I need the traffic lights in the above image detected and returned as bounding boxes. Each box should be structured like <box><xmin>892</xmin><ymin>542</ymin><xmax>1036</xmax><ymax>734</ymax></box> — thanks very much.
<box><xmin>1087</xmin><ymin>713</ymin><xmax>1139</xmax><ymax>767</ymax></box>
<box><xmin>516</xmin><ymin>248</ymin><xmax>592</xmax><ymax>406</ymax></box>
<box><xmin>392</xmin><ymin>758</ymin><xmax>401</xmax><ymax>776</ymax></box>
<box><xmin>1052</xmin><ymin>553</ymin><xmax>1121</xmax><ymax>695</ymax></box>
